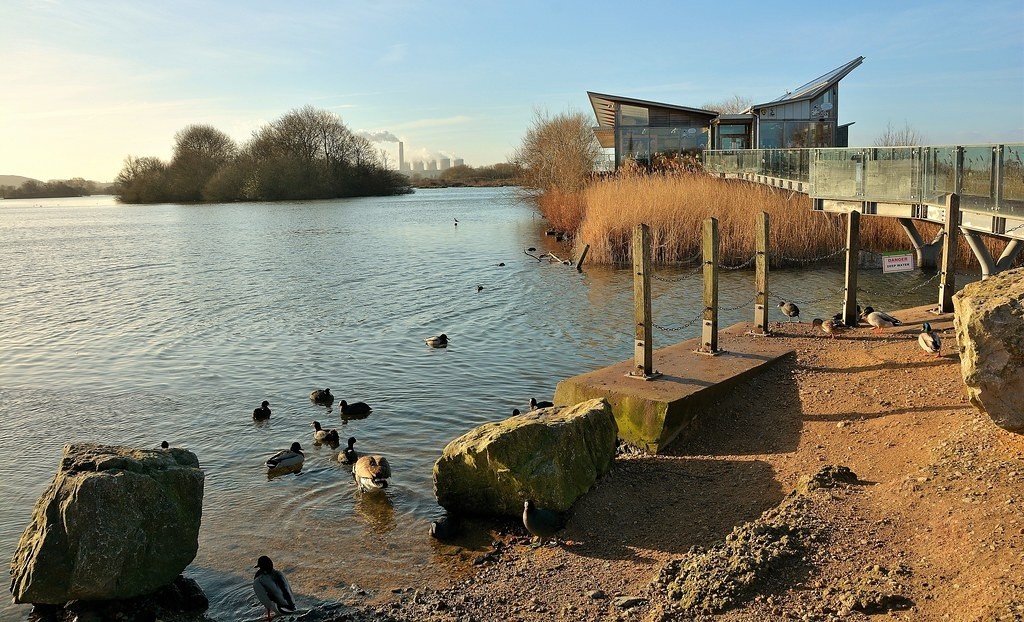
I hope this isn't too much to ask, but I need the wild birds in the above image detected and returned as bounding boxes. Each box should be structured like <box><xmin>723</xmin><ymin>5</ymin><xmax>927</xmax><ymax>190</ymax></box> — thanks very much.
<box><xmin>161</xmin><ymin>440</ymin><xmax>169</xmax><ymax>449</ymax></box>
<box><xmin>523</xmin><ymin>500</ymin><xmax>566</xmax><ymax>548</ymax></box>
<box><xmin>428</xmin><ymin>513</ymin><xmax>450</xmax><ymax>538</ymax></box>
<box><xmin>252</xmin><ymin>555</ymin><xmax>298</xmax><ymax>620</ymax></box>
<box><xmin>338</xmin><ymin>437</ymin><xmax>358</xmax><ymax>462</ymax></box>
<box><xmin>264</xmin><ymin>442</ymin><xmax>305</xmax><ymax>470</ymax></box>
<box><xmin>310</xmin><ymin>388</ymin><xmax>334</xmax><ymax>404</ymax></box>
<box><xmin>918</xmin><ymin>322</ymin><xmax>942</xmax><ymax>357</ymax></box>
<box><xmin>309</xmin><ymin>420</ymin><xmax>339</xmax><ymax>441</ymax></box>
<box><xmin>253</xmin><ymin>401</ymin><xmax>271</xmax><ymax>421</ymax></box>
<box><xmin>810</xmin><ymin>304</ymin><xmax>904</xmax><ymax>339</ymax></box>
<box><xmin>475</xmin><ymin>285</ymin><xmax>483</xmax><ymax>290</ymax></box>
<box><xmin>453</xmin><ymin>217</ymin><xmax>459</xmax><ymax>222</ymax></box>
<box><xmin>424</xmin><ymin>334</ymin><xmax>450</xmax><ymax>345</ymax></box>
<box><xmin>337</xmin><ymin>400</ymin><xmax>371</xmax><ymax>413</ymax></box>
<box><xmin>340</xmin><ymin>411</ymin><xmax>373</xmax><ymax>425</ymax></box>
<box><xmin>352</xmin><ymin>454</ymin><xmax>392</xmax><ymax>493</ymax></box>
<box><xmin>528</xmin><ymin>397</ymin><xmax>554</xmax><ymax>411</ymax></box>
<box><xmin>514</xmin><ymin>409</ymin><xmax>520</xmax><ymax>418</ymax></box>
<box><xmin>777</xmin><ymin>302</ymin><xmax>801</xmax><ymax>323</ymax></box>
